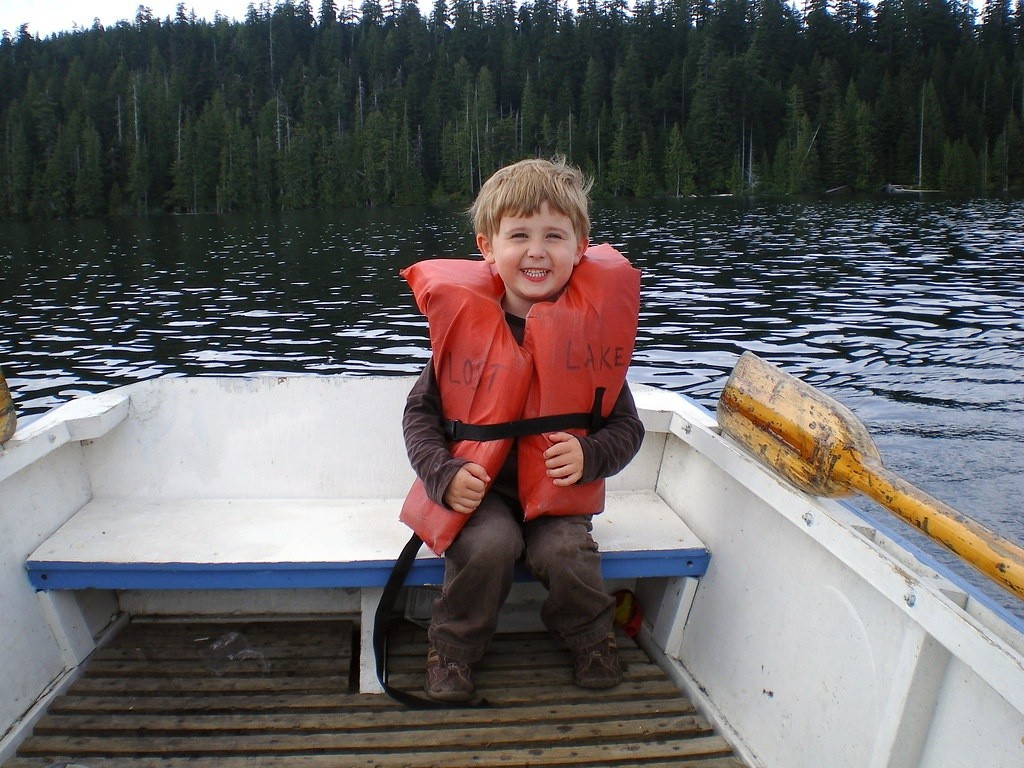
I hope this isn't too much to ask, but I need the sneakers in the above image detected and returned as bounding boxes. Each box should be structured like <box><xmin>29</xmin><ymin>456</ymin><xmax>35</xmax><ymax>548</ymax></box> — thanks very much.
<box><xmin>425</xmin><ymin>642</ymin><xmax>476</xmax><ymax>701</ymax></box>
<box><xmin>572</xmin><ymin>624</ymin><xmax>622</xmax><ymax>689</ymax></box>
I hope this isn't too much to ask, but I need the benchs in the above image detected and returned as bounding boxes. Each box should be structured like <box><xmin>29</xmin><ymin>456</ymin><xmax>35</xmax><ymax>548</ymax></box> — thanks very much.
<box><xmin>26</xmin><ymin>489</ymin><xmax>711</xmax><ymax>695</ymax></box>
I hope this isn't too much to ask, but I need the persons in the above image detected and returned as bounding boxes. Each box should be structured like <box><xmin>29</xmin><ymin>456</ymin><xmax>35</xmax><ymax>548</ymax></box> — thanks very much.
<box><xmin>399</xmin><ymin>159</ymin><xmax>646</xmax><ymax>700</ymax></box>
